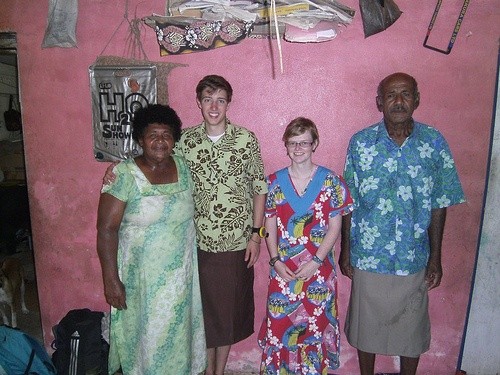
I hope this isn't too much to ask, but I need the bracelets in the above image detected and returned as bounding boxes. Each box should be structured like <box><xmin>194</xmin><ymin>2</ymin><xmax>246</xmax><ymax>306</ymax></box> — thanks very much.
<box><xmin>312</xmin><ymin>255</ymin><xmax>323</xmax><ymax>266</ymax></box>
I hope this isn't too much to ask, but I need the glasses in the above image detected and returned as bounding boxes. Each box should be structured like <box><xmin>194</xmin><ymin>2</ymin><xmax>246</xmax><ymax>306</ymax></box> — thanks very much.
<box><xmin>284</xmin><ymin>140</ymin><xmax>314</xmax><ymax>148</ymax></box>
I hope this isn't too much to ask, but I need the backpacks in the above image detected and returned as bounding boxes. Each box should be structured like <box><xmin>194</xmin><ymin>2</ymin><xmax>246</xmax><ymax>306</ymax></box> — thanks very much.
<box><xmin>50</xmin><ymin>309</ymin><xmax>110</xmax><ymax>375</ymax></box>
<box><xmin>0</xmin><ymin>324</ymin><xmax>57</xmax><ymax>375</ymax></box>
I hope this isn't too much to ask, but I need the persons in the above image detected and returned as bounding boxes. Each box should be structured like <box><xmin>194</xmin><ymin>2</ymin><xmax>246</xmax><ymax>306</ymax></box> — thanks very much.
<box><xmin>104</xmin><ymin>74</ymin><xmax>269</xmax><ymax>375</ymax></box>
<box><xmin>338</xmin><ymin>72</ymin><xmax>466</xmax><ymax>375</ymax></box>
<box><xmin>97</xmin><ymin>104</ymin><xmax>208</xmax><ymax>375</ymax></box>
<box><xmin>257</xmin><ymin>116</ymin><xmax>354</xmax><ymax>375</ymax></box>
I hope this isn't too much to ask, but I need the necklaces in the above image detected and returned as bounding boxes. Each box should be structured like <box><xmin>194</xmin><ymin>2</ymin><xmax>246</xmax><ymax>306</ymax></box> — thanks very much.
<box><xmin>294</xmin><ymin>175</ymin><xmax>310</xmax><ymax>194</ymax></box>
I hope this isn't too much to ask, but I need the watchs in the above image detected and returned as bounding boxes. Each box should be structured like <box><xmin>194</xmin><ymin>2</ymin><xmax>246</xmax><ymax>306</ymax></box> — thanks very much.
<box><xmin>269</xmin><ymin>256</ymin><xmax>279</xmax><ymax>266</ymax></box>
<box><xmin>252</xmin><ymin>226</ymin><xmax>267</xmax><ymax>238</ymax></box>
